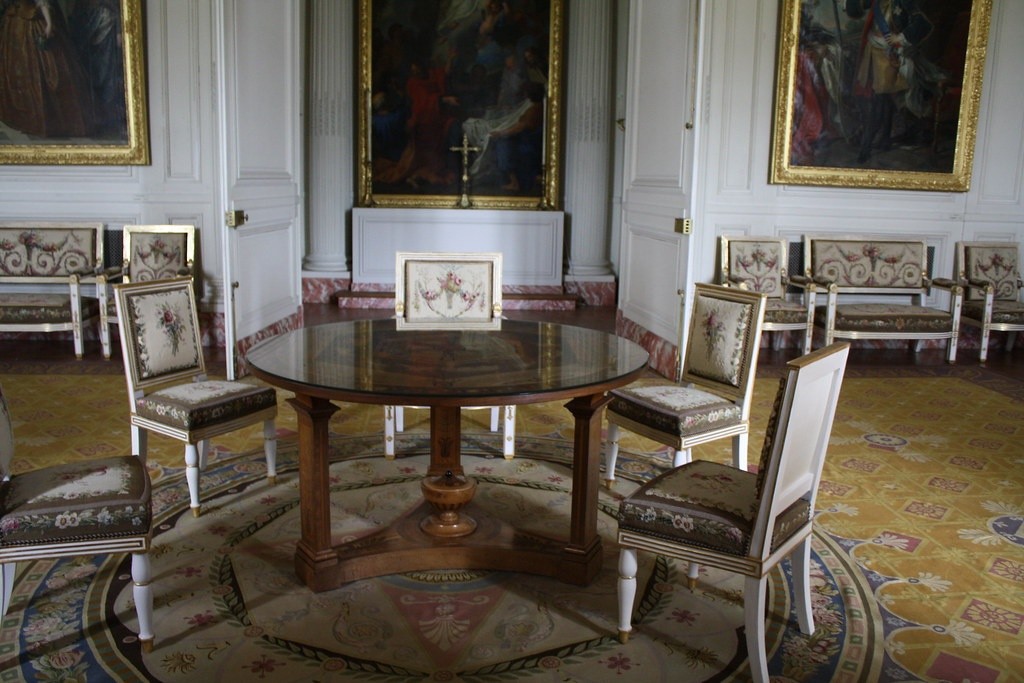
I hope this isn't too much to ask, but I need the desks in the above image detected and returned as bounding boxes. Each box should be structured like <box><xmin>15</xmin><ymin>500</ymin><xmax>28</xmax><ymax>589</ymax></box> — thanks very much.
<box><xmin>245</xmin><ymin>317</ymin><xmax>652</xmax><ymax>595</ymax></box>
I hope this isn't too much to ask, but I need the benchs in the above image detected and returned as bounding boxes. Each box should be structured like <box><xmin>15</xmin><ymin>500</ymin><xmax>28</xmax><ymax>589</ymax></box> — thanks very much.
<box><xmin>802</xmin><ymin>234</ymin><xmax>964</xmax><ymax>368</ymax></box>
<box><xmin>0</xmin><ymin>221</ymin><xmax>104</xmax><ymax>360</ymax></box>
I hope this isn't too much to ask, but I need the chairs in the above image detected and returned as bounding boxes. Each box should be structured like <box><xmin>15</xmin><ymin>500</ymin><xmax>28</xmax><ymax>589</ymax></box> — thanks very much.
<box><xmin>115</xmin><ymin>273</ymin><xmax>281</xmax><ymax>520</ymax></box>
<box><xmin>616</xmin><ymin>341</ymin><xmax>852</xmax><ymax>683</ymax></box>
<box><xmin>603</xmin><ymin>281</ymin><xmax>768</xmax><ymax>492</ymax></box>
<box><xmin>0</xmin><ymin>404</ymin><xmax>155</xmax><ymax>655</ymax></box>
<box><xmin>951</xmin><ymin>240</ymin><xmax>1024</xmax><ymax>363</ymax></box>
<box><xmin>94</xmin><ymin>224</ymin><xmax>196</xmax><ymax>360</ymax></box>
<box><xmin>720</xmin><ymin>232</ymin><xmax>817</xmax><ymax>358</ymax></box>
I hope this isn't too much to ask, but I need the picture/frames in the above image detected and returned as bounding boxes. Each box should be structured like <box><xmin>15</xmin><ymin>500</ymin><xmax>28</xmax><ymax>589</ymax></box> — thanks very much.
<box><xmin>395</xmin><ymin>251</ymin><xmax>503</xmax><ymax>333</ymax></box>
<box><xmin>0</xmin><ymin>0</ymin><xmax>152</xmax><ymax>168</ymax></box>
<box><xmin>768</xmin><ymin>0</ymin><xmax>994</xmax><ymax>194</ymax></box>
<box><xmin>354</xmin><ymin>0</ymin><xmax>566</xmax><ymax>213</ymax></box>
